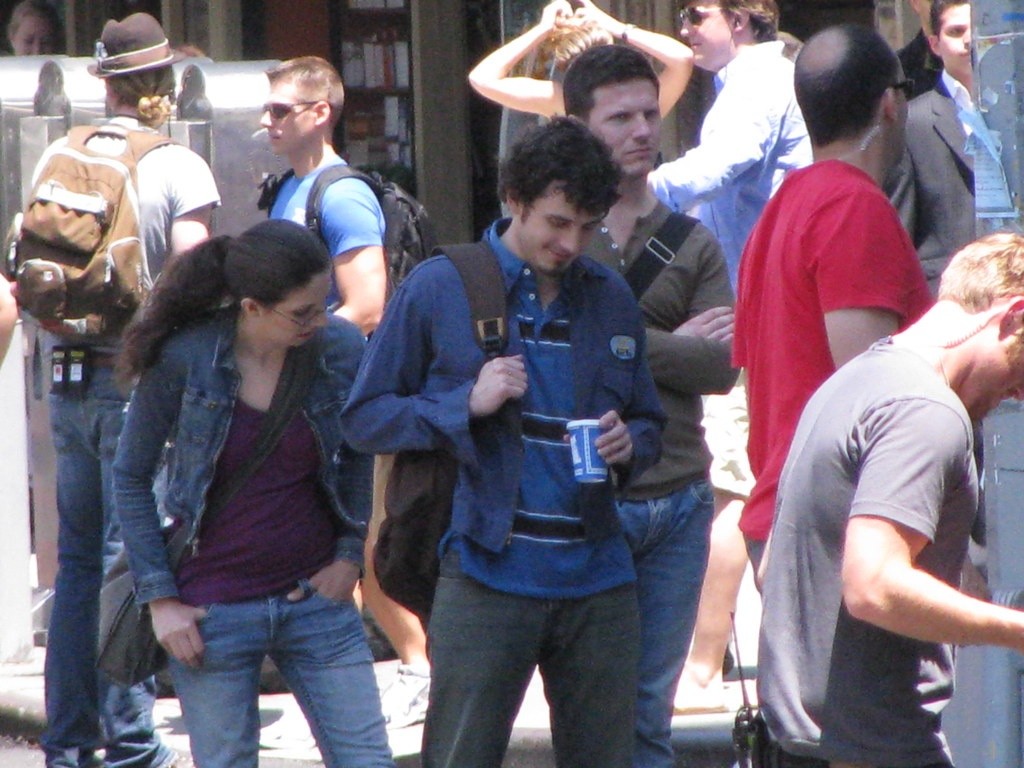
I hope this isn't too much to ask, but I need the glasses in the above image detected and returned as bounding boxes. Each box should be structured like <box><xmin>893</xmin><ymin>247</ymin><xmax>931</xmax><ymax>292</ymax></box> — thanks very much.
<box><xmin>679</xmin><ymin>6</ymin><xmax>729</xmax><ymax>26</ymax></box>
<box><xmin>264</xmin><ymin>295</ymin><xmax>344</xmax><ymax>328</ymax></box>
<box><xmin>263</xmin><ymin>99</ymin><xmax>330</xmax><ymax>119</ymax></box>
<box><xmin>889</xmin><ymin>79</ymin><xmax>915</xmax><ymax>96</ymax></box>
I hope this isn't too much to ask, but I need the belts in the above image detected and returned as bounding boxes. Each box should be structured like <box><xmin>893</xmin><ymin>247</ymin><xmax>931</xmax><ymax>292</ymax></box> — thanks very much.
<box><xmin>758</xmin><ymin>741</ymin><xmax>830</xmax><ymax>768</ymax></box>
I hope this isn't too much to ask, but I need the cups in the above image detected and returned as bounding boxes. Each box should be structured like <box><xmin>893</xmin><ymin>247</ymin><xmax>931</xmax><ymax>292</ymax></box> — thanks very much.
<box><xmin>567</xmin><ymin>419</ymin><xmax>608</xmax><ymax>483</ymax></box>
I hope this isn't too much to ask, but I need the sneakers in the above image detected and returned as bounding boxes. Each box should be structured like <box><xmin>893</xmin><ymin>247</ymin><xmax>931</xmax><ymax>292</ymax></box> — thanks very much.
<box><xmin>381</xmin><ymin>665</ymin><xmax>430</xmax><ymax>727</ymax></box>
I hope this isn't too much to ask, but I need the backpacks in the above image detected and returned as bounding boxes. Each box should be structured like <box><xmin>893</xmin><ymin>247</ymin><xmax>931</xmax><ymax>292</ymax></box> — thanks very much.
<box><xmin>4</xmin><ymin>126</ymin><xmax>178</xmax><ymax>344</ymax></box>
<box><xmin>374</xmin><ymin>237</ymin><xmax>509</xmax><ymax>617</ymax></box>
<box><xmin>267</xmin><ymin>169</ymin><xmax>436</xmax><ymax>317</ymax></box>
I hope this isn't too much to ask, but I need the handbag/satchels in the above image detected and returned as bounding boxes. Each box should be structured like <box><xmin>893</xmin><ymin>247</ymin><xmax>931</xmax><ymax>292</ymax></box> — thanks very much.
<box><xmin>96</xmin><ymin>546</ymin><xmax>173</xmax><ymax>687</ymax></box>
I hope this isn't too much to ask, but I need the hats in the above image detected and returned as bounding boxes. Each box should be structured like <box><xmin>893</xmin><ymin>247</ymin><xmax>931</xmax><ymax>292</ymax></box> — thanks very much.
<box><xmin>86</xmin><ymin>12</ymin><xmax>184</xmax><ymax>78</ymax></box>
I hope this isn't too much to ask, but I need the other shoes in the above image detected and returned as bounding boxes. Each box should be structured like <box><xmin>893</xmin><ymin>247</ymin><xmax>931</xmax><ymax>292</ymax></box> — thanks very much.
<box><xmin>673</xmin><ymin>662</ymin><xmax>730</xmax><ymax>715</ymax></box>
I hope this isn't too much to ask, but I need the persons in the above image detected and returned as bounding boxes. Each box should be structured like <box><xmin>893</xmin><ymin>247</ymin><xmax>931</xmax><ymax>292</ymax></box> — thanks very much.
<box><xmin>731</xmin><ymin>22</ymin><xmax>939</xmax><ymax>569</ymax></box>
<box><xmin>644</xmin><ymin>0</ymin><xmax>812</xmax><ymax>715</ymax></box>
<box><xmin>113</xmin><ymin>218</ymin><xmax>396</xmax><ymax>768</ymax></box>
<box><xmin>465</xmin><ymin>0</ymin><xmax>693</xmax><ymax>119</ymax></box>
<box><xmin>265</xmin><ymin>57</ymin><xmax>431</xmax><ymax>732</ymax></box>
<box><xmin>751</xmin><ymin>233</ymin><xmax>1024</xmax><ymax>768</ymax></box>
<box><xmin>17</xmin><ymin>13</ymin><xmax>222</xmax><ymax>768</ymax></box>
<box><xmin>897</xmin><ymin>0</ymin><xmax>975</xmax><ymax>281</ymax></box>
<box><xmin>8</xmin><ymin>0</ymin><xmax>60</xmax><ymax>55</ymax></box>
<box><xmin>561</xmin><ymin>45</ymin><xmax>740</xmax><ymax>768</ymax></box>
<box><xmin>340</xmin><ymin>119</ymin><xmax>663</xmax><ymax>768</ymax></box>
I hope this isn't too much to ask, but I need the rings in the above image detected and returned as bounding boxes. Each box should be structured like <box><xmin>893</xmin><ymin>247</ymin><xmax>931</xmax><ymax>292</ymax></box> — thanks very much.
<box><xmin>508</xmin><ymin>368</ymin><xmax>512</xmax><ymax>375</ymax></box>
